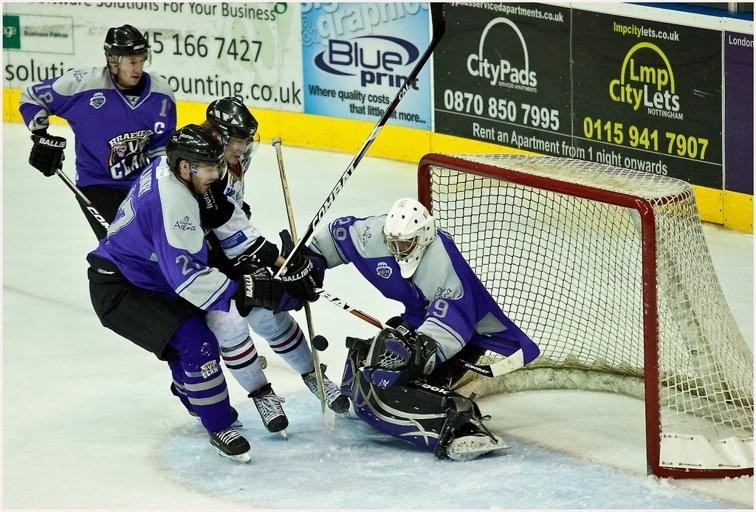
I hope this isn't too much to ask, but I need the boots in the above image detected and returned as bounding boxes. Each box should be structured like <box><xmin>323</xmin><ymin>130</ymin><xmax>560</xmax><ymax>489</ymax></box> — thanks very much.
<box><xmin>207</xmin><ymin>429</ymin><xmax>251</xmax><ymax>455</ymax></box>
<box><xmin>171</xmin><ymin>382</ymin><xmax>238</xmax><ymax>425</ymax></box>
<box><xmin>247</xmin><ymin>382</ymin><xmax>289</xmax><ymax>433</ymax></box>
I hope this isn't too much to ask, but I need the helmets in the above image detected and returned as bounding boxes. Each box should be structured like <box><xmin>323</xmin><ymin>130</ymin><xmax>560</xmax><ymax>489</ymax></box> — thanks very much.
<box><xmin>383</xmin><ymin>197</ymin><xmax>438</xmax><ymax>280</ymax></box>
<box><xmin>103</xmin><ymin>24</ymin><xmax>151</xmax><ymax>71</ymax></box>
<box><xmin>165</xmin><ymin>123</ymin><xmax>229</xmax><ymax>181</ymax></box>
<box><xmin>205</xmin><ymin>97</ymin><xmax>260</xmax><ymax>160</ymax></box>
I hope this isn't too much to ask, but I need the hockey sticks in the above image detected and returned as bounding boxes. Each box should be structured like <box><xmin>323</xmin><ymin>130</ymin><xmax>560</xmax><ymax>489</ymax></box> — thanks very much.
<box><xmin>271</xmin><ymin>135</ymin><xmax>336</xmax><ymax>428</ymax></box>
<box><xmin>271</xmin><ymin>2</ymin><xmax>444</xmax><ymax>279</ymax></box>
<box><xmin>313</xmin><ymin>288</ymin><xmax>524</xmax><ymax>377</ymax></box>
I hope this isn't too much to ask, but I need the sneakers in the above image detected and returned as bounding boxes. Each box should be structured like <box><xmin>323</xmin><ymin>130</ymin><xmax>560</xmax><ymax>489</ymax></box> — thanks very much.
<box><xmin>446</xmin><ymin>427</ymin><xmax>494</xmax><ymax>462</ymax></box>
<box><xmin>301</xmin><ymin>364</ymin><xmax>350</xmax><ymax>414</ymax></box>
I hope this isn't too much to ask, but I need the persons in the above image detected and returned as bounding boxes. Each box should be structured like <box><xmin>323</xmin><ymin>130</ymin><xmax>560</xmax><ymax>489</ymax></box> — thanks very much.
<box><xmin>200</xmin><ymin>96</ymin><xmax>350</xmax><ymax>434</ymax></box>
<box><xmin>272</xmin><ymin>198</ymin><xmax>540</xmax><ymax>460</ymax></box>
<box><xmin>20</xmin><ymin>23</ymin><xmax>177</xmax><ymax>242</ymax></box>
<box><xmin>85</xmin><ymin>124</ymin><xmax>312</xmax><ymax>455</ymax></box>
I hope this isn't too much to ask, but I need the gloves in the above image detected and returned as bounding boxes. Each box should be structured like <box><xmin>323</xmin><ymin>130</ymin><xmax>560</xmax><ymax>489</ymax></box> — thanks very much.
<box><xmin>235</xmin><ymin>265</ymin><xmax>283</xmax><ymax>319</ymax></box>
<box><xmin>277</xmin><ymin>254</ymin><xmax>326</xmax><ymax>303</ymax></box>
<box><xmin>28</xmin><ymin>134</ymin><xmax>66</xmax><ymax>177</ymax></box>
